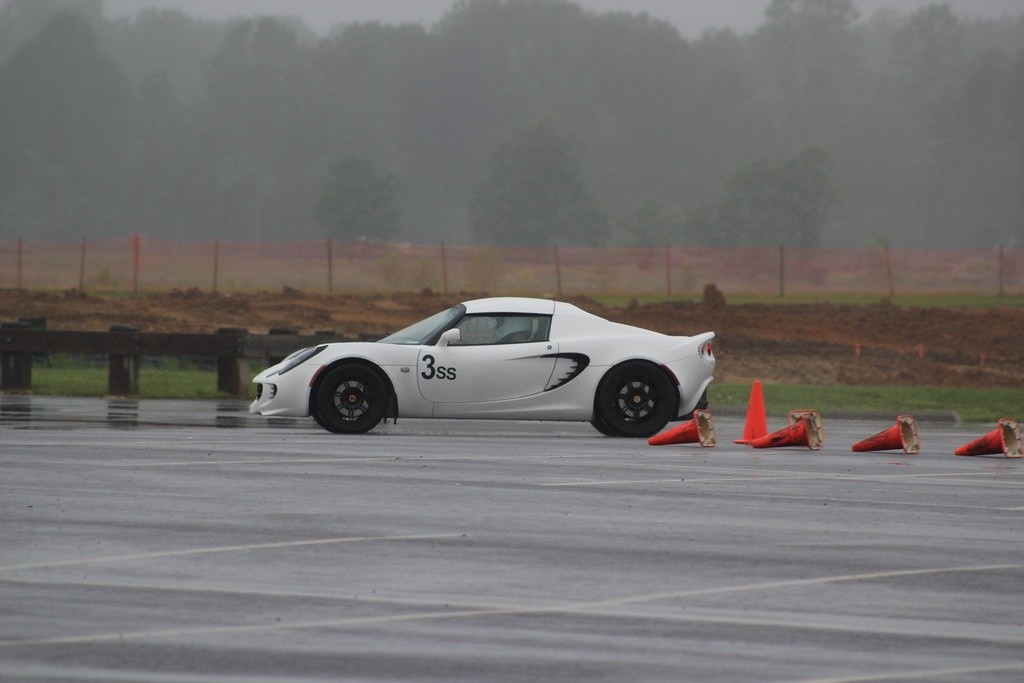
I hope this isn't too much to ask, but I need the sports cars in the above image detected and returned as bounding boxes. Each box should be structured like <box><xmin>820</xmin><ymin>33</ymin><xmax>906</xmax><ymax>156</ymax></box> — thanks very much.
<box><xmin>249</xmin><ymin>297</ymin><xmax>717</xmax><ymax>438</ymax></box>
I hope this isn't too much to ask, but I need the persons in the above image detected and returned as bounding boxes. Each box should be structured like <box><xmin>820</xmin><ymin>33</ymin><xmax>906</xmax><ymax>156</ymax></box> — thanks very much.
<box><xmin>493</xmin><ymin>316</ymin><xmax>533</xmax><ymax>343</ymax></box>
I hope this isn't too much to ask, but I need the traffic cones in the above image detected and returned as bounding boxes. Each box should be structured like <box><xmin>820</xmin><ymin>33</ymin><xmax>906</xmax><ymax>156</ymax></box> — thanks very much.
<box><xmin>752</xmin><ymin>414</ymin><xmax>820</xmax><ymax>452</ymax></box>
<box><xmin>733</xmin><ymin>381</ymin><xmax>768</xmax><ymax>443</ymax></box>
<box><xmin>648</xmin><ymin>410</ymin><xmax>716</xmax><ymax>447</ymax></box>
<box><xmin>788</xmin><ymin>409</ymin><xmax>824</xmax><ymax>447</ymax></box>
<box><xmin>953</xmin><ymin>418</ymin><xmax>1024</xmax><ymax>459</ymax></box>
<box><xmin>851</xmin><ymin>415</ymin><xmax>920</xmax><ymax>454</ymax></box>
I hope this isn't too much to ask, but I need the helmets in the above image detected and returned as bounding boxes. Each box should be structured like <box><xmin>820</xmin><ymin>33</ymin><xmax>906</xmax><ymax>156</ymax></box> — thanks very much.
<box><xmin>493</xmin><ymin>316</ymin><xmax>533</xmax><ymax>343</ymax></box>
